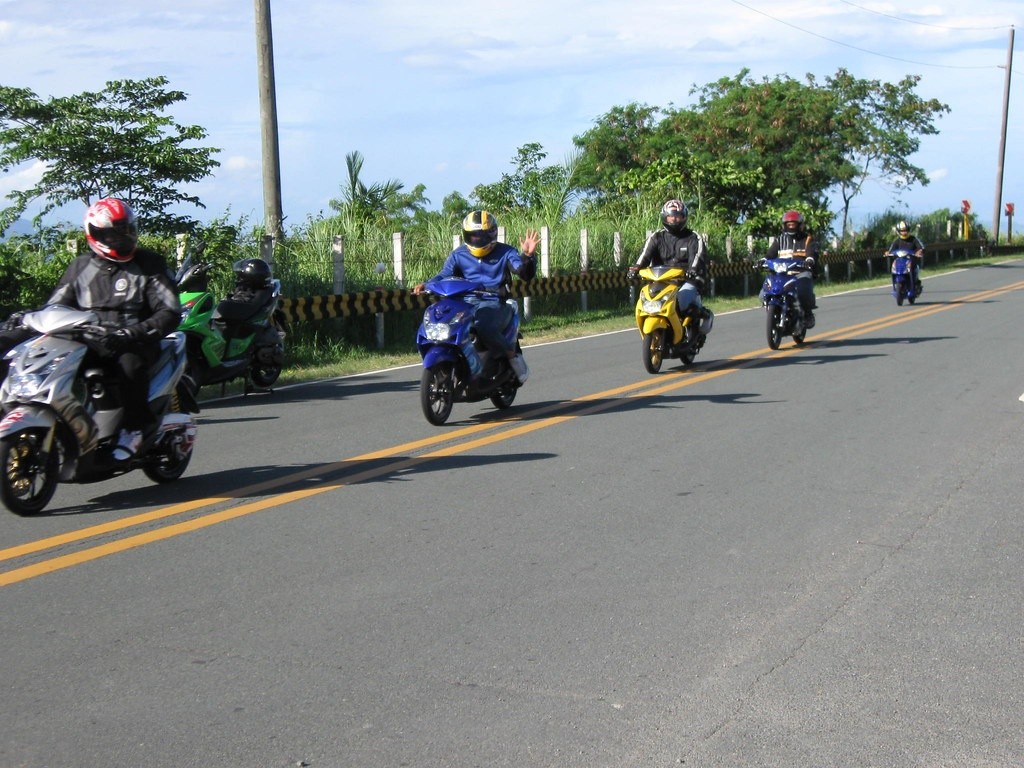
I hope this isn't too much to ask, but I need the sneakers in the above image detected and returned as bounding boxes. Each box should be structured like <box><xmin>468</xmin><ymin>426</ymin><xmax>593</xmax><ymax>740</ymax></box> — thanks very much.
<box><xmin>806</xmin><ymin>310</ymin><xmax>816</xmax><ymax>327</ymax></box>
<box><xmin>509</xmin><ymin>354</ymin><xmax>531</xmax><ymax>384</ymax></box>
<box><xmin>111</xmin><ymin>429</ymin><xmax>144</xmax><ymax>461</ymax></box>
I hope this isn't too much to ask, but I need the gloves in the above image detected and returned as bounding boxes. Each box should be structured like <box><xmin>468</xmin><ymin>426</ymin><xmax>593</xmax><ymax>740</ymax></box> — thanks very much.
<box><xmin>685</xmin><ymin>267</ymin><xmax>698</xmax><ymax>278</ymax></box>
<box><xmin>626</xmin><ymin>264</ymin><xmax>640</xmax><ymax>278</ymax></box>
<box><xmin>806</xmin><ymin>259</ymin><xmax>814</xmax><ymax>269</ymax></box>
<box><xmin>0</xmin><ymin>308</ymin><xmax>31</xmax><ymax>332</ymax></box>
<box><xmin>99</xmin><ymin>328</ymin><xmax>134</xmax><ymax>355</ymax></box>
<box><xmin>756</xmin><ymin>259</ymin><xmax>765</xmax><ymax>272</ymax></box>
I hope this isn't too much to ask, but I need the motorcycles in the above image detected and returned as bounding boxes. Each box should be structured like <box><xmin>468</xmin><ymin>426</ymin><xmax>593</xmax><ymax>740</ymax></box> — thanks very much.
<box><xmin>633</xmin><ymin>265</ymin><xmax>715</xmax><ymax>374</ymax></box>
<box><xmin>0</xmin><ymin>303</ymin><xmax>200</xmax><ymax>517</ymax></box>
<box><xmin>172</xmin><ymin>241</ymin><xmax>286</xmax><ymax>398</ymax></box>
<box><xmin>752</xmin><ymin>258</ymin><xmax>807</xmax><ymax>350</ymax></box>
<box><xmin>883</xmin><ymin>248</ymin><xmax>923</xmax><ymax>305</ymax></box>
<box><xmin>409</xmin><ymin>275</ymin><xmax>524</xmax><ymax>426</ymax></box>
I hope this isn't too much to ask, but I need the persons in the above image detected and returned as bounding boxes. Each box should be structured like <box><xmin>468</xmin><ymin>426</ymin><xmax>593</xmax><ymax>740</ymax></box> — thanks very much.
<box><xmin>414</xmin><ymin>210</ymin><xmax>542</xmax><ymax>384</ymax></box>
<box><xmin>756</xmin><ymin>210</ymin><xmax>819</xmax><ymax>328</ymax></box>
<box><xmin>2</xmin><ymin>198</ymin><xmax>182</xmax><ymax>461</ymax></box>
<box><xmin>884</xmin><ymin>220</ymin><xmax>925</xmax><ymax>295</ymax></box>
<box><xmin>626</xmin><ymin>200</ymin><xmax>714</xmax><ymax>334</ymax></box>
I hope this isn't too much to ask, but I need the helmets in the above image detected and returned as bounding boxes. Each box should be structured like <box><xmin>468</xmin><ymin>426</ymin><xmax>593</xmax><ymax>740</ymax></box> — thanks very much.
<box><xmin>895</xmin><ymin>221</ymin><xmax>911</xmax><ymax>240</ymax></box>
<box><xmin>231</xmin><ymin>257</ymin><xmax>272</xmax><ymax>290</ymax></box>
<box><xmin>462</xmin><ymin>210</ymin><xmax>498</xmax><ymax>257</ymax></box>
<box><xmin>83</xmin><ymin>197</ymin><xmax>140</xmax><ymax>263</ymax></box>
<box><xmin>660</xmin><ymin>199</ymin><xmax>688</xmax><ymax>234</ymax></box>
<box><xmin>781</xmin><ymin>211</ymin><xmax>805</xmax><ymax>238</ymax></box>
